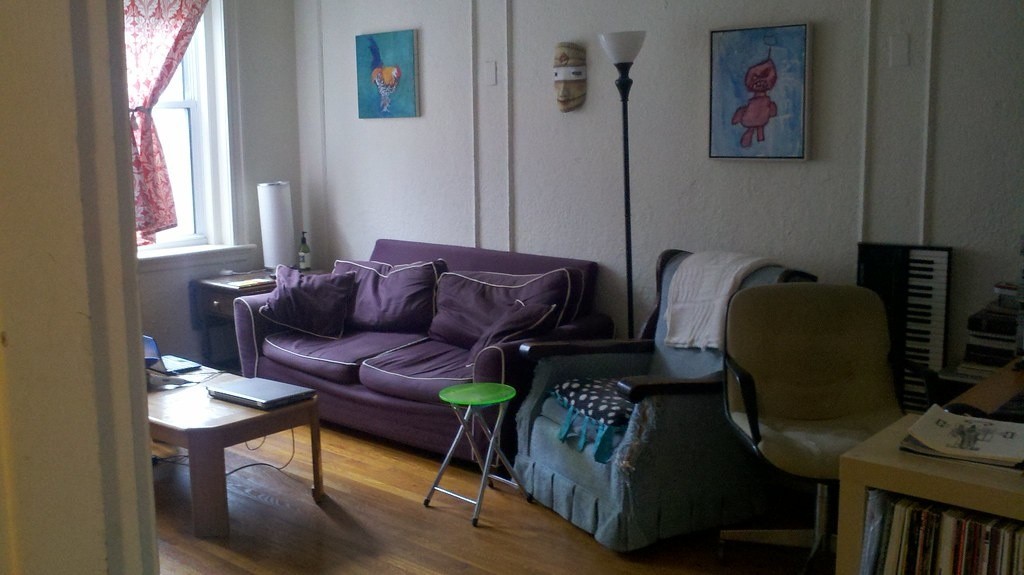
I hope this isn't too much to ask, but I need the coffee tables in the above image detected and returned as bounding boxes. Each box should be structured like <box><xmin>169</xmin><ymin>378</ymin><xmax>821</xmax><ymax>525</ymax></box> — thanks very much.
<box><xmin>147</xmin><ymin>362</ymin><xmax>324</xmax><ymax>537</ymax></box>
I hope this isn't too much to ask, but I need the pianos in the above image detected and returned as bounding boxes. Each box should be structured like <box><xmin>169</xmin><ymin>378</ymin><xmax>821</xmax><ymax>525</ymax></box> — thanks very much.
<box><xmin>852</xmin><ymin>241</ymin><xmax>953</xmax><ymax>420</ymax></box>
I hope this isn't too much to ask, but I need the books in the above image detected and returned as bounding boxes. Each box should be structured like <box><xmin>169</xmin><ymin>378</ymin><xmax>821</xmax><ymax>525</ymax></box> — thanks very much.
<box><xmin>228</xmin><ymin>279</ymin><xmax>274</xmax><ymax>289</ymax></box>
<box><xmin>863</xmin><ymin>491</ymin><xmax>1024</xmax><ymax>575</ymax></box>
<box><xmin>898</xmin><ymin>404</ymin><xmax>1024</xmax><ymax>468</ymax></box>
<box><xmin>957</xmin><ymin>281</ymin><xmax>1024</xmax><ymax>377</ymax></box>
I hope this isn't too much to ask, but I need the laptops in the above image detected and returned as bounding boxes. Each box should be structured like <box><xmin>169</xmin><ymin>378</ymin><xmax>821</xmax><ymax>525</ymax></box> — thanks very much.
<box><xmin>207</xmin><ymin>377</ymin><xmax>316</xmax><ymax>409</ymax></box>
<box><xmin>143</xmin><ymin>334</ymin><xmax>200</xmax><ymax>374</ymax></box>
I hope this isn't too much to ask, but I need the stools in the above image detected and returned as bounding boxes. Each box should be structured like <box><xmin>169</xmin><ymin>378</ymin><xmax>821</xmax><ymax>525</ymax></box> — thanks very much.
<box><xmin>423</xmin><ymin>383</ymin><xmax>534</xmax><ymax>524</ymax></box>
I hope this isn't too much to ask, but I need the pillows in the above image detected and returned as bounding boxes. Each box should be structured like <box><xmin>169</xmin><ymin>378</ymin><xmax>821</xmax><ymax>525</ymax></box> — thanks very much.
<box><xmin>547</xmin><ymin>377</ymin><xmax>632</xmax><ymax>464</ymax></box>
<box><xmin>467</xmin><ymin>300</ymin><xmax>557</xmax><ymax>367</ymax></box>
<box><xmin>257</xmin><ymin>264</ymin><xmax>357</xmax><ymax>341</ymax></box>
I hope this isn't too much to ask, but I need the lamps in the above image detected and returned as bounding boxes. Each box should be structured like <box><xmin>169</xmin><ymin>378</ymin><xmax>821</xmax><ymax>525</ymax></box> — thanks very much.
<box><xmin>258</xmin><ymin>182</ymin><xmax>298</xmax><ymax>277</ymax></box>
<box><xmin>597</xmin><ymin>29</ymin><xmax>646</xmax><ymax>339</ymax></box>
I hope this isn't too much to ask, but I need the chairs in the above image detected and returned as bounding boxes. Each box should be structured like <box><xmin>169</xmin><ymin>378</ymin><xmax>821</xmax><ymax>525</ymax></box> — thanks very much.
<box><xmin>713</xmin><ymin>282</ymin><xmax>907</xmax><ymax>575</ymax></box>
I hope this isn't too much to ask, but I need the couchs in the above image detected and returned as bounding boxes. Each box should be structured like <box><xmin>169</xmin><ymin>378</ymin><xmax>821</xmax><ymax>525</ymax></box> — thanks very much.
<box><xmin>509</xmin><ymin>249</ymin><xmax>816</xmax><ymax>557</ymax></box>
<box><xmin>232</xmin><ymin>238</ymin><xmax>614</xmax><ymax>470</ymax></box>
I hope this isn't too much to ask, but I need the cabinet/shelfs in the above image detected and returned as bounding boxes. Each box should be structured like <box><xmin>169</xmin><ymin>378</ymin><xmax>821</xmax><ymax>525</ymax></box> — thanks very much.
<box><xmin>195</xmin><ymin>270</ymin><xmax>327</xmax><ymax>366</ymax></box>
<box><xmin>835</xmin><ymin>412</ymin><xmax>1024</xmax><ymax>575</ymax></box>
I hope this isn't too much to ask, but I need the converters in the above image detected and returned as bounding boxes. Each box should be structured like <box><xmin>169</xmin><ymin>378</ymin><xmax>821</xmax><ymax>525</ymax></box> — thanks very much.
<box><xmin>152</xmin><ymin>455</ymin><xmax>159</xmax><ymax>465</ymax></box>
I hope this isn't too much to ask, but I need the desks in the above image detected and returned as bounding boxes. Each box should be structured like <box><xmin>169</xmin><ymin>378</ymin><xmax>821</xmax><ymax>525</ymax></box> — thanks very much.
<box><xmin>941</xmin><ymin>357</ymin><xmax>1024</xmax><ymax>422</ymax></box>
